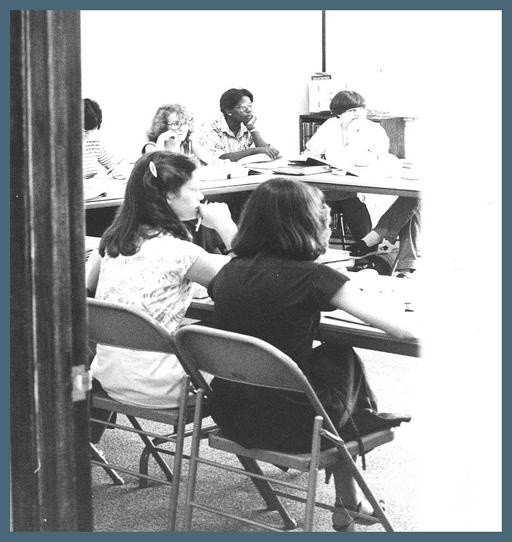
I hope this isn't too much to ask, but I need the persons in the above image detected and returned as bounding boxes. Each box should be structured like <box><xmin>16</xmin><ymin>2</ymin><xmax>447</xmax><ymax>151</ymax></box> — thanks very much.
<box><xmin>85</xmin><ymin>149</ymin><xmax>245</xmax><ymax>410</ymax></box>
<box><xmin>141</xmin><ymin>103</ymin><xmax>207</xmax><ymax>169</ymax></box>
<box><xmin>83</xmin><ymin>97</ymin><xmax>134</xmax><ymax>204</ymax></box>
<box><xmin>200</xmin><ymin>87</ymin><xmax>281</xmax><ymax>225</ymax></box>
<box><xmin>302</xmin><ymin>90</ymin><xmax>390</xmax><ymax>242</ymax></box>
<box><xmin>346</xmin><ymin>196</ymin><xmax>422</xmax><ymax>280</ymax></box>
<box><xmin>207</xmin><ymin>180</ymin><xmax>418</xmax><ymax>534</ymax></box>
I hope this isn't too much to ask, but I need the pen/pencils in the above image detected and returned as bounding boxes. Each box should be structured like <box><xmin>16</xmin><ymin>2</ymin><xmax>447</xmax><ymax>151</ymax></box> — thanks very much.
<box><xmin>195</xmin><ymin>200</ymin><xmax>208</xmax><ymax>232</ymax></box>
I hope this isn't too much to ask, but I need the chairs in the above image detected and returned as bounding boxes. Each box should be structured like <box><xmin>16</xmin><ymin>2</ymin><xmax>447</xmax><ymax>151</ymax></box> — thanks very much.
<box><xmin>175</xmin><ymin>324</ymin><xmax>396</xmax><ymax>531</ymax></box>
<box><xmin>84</xmin><ymin>298</ymin><xmax>275</xmax><ymax>531</ymax></box>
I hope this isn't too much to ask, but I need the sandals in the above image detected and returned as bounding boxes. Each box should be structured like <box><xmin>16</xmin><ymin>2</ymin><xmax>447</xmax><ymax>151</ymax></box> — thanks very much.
<box><xmin>333</xmin><ymin>501</ymin><xmax>385</xmax><ymax>532</ymax></box>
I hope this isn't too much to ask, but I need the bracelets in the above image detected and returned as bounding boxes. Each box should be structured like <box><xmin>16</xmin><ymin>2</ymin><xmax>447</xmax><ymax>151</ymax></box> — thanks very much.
<box><xmin>250</xmin><ymin>129</ymin><xmax>257</xmax><ymax>132</ymax></box>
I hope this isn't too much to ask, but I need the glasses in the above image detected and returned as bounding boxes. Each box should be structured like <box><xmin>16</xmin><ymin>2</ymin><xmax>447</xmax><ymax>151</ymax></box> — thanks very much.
<box><xmin>234</xmin><ymin>102</ymin><xmax>253</xmax><ymax>113</ymax></box>
<box><xmin>167</xmin><ymin>119</ymin><xmax>192</xmax><ymax>132</ymax></box>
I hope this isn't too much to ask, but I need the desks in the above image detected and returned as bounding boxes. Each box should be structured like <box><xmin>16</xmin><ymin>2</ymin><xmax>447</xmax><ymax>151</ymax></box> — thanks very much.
<box><xmin>83</xmin><ymin>161</ymin><xmax>421</xmax><ymax>272</ymax></box>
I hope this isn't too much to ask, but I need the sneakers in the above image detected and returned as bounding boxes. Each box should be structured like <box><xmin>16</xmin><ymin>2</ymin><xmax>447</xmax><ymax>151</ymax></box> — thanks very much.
<box><xmin>345</xmin><ymin>238</ymin><xmax>378</xmax><ymax>259</ymax></box>
